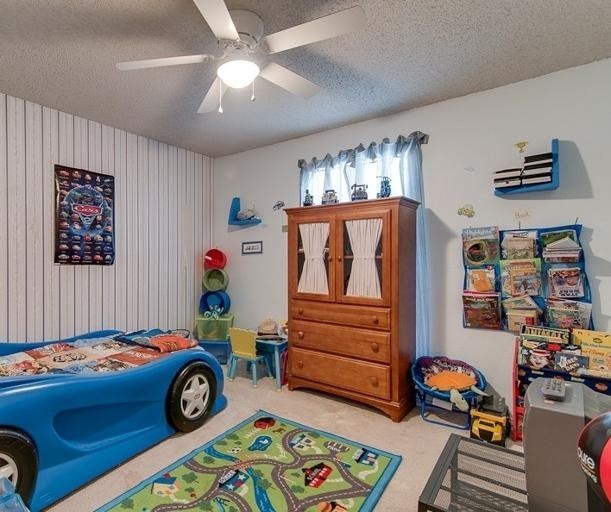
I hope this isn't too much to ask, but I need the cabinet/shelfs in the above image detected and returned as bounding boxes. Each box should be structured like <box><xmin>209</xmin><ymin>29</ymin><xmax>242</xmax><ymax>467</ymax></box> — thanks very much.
<box><xmin>510</xmin><ymin>334</ymin><xmax>611</xmax><ymax>442</ymax></box>
<box><xmin>283</xmin><ymin>198</ymin><xmax>421</xmax><ymax>422</ymax></box>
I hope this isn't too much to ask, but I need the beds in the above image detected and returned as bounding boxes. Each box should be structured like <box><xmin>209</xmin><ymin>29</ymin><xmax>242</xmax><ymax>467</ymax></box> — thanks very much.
<box><xmin>0</xmin><ymin>329</ymin><xmax>228</xmax><ymax>512</ymax></box>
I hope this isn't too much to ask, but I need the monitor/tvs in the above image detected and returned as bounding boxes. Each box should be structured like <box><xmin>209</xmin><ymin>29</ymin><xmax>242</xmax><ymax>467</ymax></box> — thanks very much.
<box><xmin>523</xmin><ymin>377</ymin><xmax>611</xmax><ymax>512</ymax></box>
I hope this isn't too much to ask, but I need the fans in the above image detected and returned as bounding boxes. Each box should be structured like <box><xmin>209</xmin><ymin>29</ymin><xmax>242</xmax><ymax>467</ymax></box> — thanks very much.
<box><xmin>113</xmin><ymin>2</ymin><xmax>368</xmax><ymax>116</ymax></box>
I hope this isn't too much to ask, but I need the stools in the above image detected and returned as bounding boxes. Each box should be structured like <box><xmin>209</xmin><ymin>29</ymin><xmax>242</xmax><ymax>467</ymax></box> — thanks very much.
<box><xmin>412</xmin><ymin>354</ymin><xmax>485</xmax><ymax>430</ymax></box>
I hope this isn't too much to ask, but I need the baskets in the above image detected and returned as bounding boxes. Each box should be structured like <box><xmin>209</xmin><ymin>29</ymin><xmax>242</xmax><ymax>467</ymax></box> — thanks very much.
<box><xmin>199</xmin><ymin>340</ymin><xmax>232</xmax><ymax>364</ymax></box>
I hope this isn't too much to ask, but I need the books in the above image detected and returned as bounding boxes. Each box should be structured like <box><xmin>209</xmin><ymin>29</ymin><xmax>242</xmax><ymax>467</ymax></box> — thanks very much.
<box><xmin>461</xmin><ymin>226</ymin><xmax>594</xmax><ymax>333</ymax></box>
<box><xmin>493</xmin><ymin>151</ymin><xmax>555</xmax><ymax>189</ymax></box>
<box><xmin>514</xmin><ymin>324</ymin><xmax>611</xmax><ymax>435</ymax></box>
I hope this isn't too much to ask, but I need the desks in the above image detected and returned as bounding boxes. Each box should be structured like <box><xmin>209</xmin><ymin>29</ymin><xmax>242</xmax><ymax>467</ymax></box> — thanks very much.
<box><xmin>249</xmin><ymin>329</ymin><xmax>287</xmax><ymax>391</ymax></box>
<box><xmin>418</xmin><ymin>432</ymin><xmax>528</xmax><ymax>510</ymax></box>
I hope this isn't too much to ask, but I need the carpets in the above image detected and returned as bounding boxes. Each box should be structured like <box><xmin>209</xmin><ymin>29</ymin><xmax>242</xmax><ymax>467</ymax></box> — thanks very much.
<box><xmin>97</xmin><ymin>410</ymin><xmax>403</xmax><ymax>510</ymax></box>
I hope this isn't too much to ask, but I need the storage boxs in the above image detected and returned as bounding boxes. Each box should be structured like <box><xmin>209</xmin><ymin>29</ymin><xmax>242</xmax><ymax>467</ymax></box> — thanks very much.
<box><xmin>197</xmin><ymin>337</ymin><xmax>232</xmax><ymax>365</ymax></box>
<box><xmin>571</xmin><ymin>326</ymin><xmax>611</xmax><ymax>378</ymax></box>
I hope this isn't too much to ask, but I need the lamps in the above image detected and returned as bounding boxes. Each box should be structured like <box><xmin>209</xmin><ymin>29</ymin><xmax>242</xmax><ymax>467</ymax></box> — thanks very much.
<box><xmin>215</xmin><ymin>52</ymin><xmax>260</xmax><ymax>91</ymax></box>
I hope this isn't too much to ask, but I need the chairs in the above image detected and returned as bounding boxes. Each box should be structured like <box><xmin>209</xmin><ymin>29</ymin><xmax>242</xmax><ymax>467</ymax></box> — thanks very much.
<box><xmin>227</xmin><ymin>327</ymin><xmax>271</xmax><ymax>388</ymax></box>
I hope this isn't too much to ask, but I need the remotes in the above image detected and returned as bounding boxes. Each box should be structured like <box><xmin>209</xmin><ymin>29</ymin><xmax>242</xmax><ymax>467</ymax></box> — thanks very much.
<box><xmin>542</xmin><ymin>377</ymin><xmax>566</xmax><ymax>400</ymax></box>
